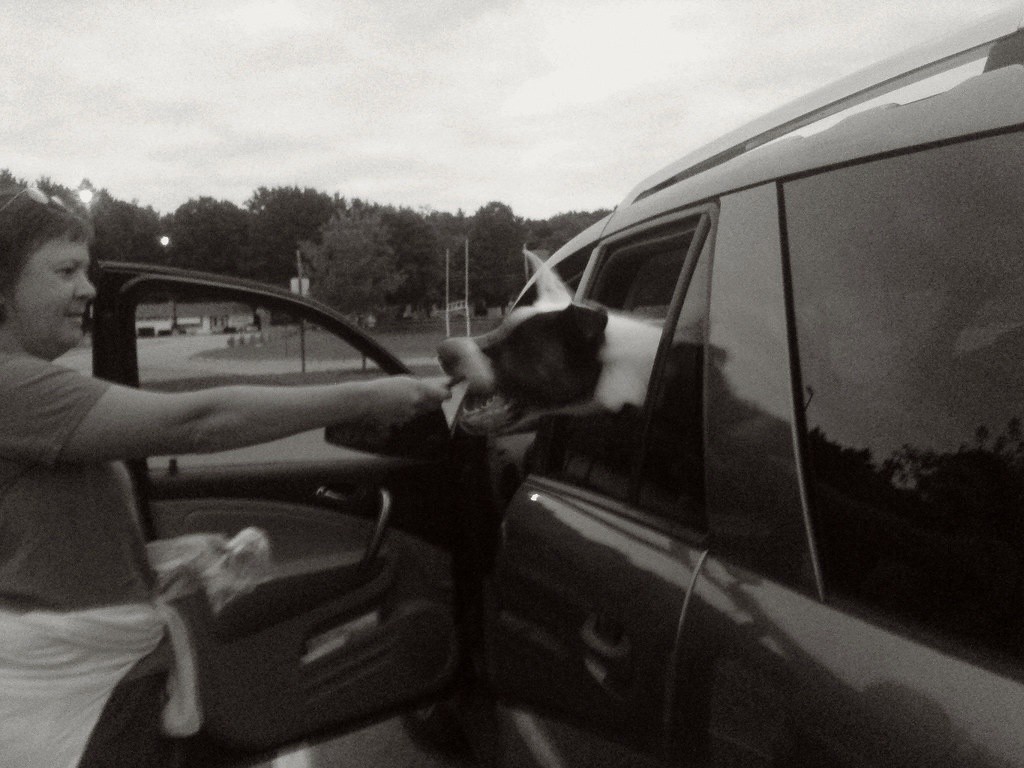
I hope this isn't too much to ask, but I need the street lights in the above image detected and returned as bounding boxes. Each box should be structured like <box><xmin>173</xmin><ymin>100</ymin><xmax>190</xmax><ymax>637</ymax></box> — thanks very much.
<box><xmin>161</xmin><ymin>236</ymin><xmax>177</xmax><ymax>330</ymax></box>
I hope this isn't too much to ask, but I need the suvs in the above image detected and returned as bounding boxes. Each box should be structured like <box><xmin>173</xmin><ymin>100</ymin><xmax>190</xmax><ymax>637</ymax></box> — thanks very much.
<box><xmin>94</xmin><ymin>1</ymin><xmax>1023</xmax><ymax>768</ymax></box>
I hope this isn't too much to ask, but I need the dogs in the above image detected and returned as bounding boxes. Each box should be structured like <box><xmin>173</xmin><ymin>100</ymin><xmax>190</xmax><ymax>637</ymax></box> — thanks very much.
<box><xmin>438</xmin><ymin>250</ymin><xmax>665</xmax><ymax>439</ymax></box>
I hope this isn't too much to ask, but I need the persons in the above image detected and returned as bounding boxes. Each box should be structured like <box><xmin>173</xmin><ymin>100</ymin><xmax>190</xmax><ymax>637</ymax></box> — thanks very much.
<box><xmin>0</xmin><ymin>186</ymin><xmax>451</xmax><ymax>768</ymax></box>
